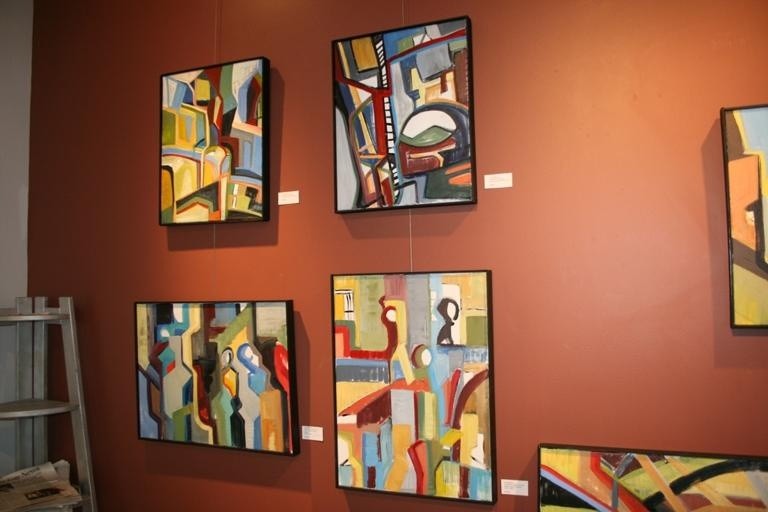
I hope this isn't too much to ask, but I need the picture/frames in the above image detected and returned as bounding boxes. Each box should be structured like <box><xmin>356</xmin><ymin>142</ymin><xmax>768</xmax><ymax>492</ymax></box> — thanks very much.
<box><xmin>329</xmin><ymin>15</ymin><xmax>478</xmax><ymax>215</ymax></box>
<box><xmin>536</xmin><ymin>443</ymin><xmax>768</xmax><ymax>511</ymax></box>
<box><xmin>158</xmin><ymin>56</ymin><xmax>271</xmax><ymax>225</ymax></box>
<box><xmin>132</xmin><ymin>298</ymin><xmax>299</xmax><ymax>456</ymax></box>
<box><xmin>719</xmin><ymin>103</ymin><xmax>768</xmax><ymax>330</ymax></box>
<box><xmin>330</xmin><ymin>269</ymin><xmax>498</xmax><ymax>506</ymax></box>
